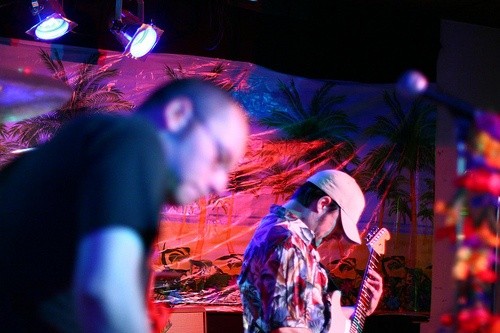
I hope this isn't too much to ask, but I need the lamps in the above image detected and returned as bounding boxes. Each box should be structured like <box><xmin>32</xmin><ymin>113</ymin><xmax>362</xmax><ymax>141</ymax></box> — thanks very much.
<box><xmin>110</xmin><ymin>1</ymin><xmax>165</xmax><ymax>65</ymax></box>
<box><xmin>25</xmin><ymin>1</ymin><xmax>77</xmax><ymax>43</ymax></box>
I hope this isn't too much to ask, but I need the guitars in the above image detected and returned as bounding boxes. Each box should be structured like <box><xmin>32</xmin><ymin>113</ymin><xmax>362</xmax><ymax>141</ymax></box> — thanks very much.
<box><xmin>326</xmin><ymin>225</ymin><xmax>390</xmax><ymax>333</ymax></box>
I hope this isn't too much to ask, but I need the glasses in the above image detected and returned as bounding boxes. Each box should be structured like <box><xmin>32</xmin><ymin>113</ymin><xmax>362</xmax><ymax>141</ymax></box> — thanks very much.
<box><xmin>193</xmin><ymin>106</ymin><xmax>229</xmax><ymax>174</ymax></box>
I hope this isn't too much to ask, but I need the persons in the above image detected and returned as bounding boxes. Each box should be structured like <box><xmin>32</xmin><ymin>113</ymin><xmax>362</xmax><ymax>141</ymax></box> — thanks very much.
<box><xmin>0</xmin><ymin>76</ymin><xmax>250</xmax><ymax>331</ymax></box>
<box><xmin>239</xmin><ymin>167</ymin><xmax>385</xmax><ymax>333</ymax></box>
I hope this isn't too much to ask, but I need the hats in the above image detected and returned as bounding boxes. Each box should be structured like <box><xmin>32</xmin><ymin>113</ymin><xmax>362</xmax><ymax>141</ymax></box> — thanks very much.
<box><xmin>307</xmin><ymin>166</ymin><xmax>364</xmax><ymax>246</ymax></box>
